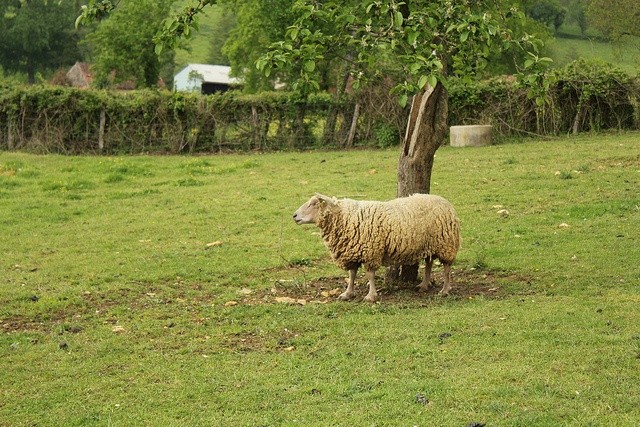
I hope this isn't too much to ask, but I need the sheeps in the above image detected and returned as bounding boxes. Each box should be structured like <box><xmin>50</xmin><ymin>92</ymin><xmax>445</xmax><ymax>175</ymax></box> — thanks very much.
<box><xmin>293</xmin><ymin>193</ymin><xmax>461</xmax><ymax>302</ymax></box>
